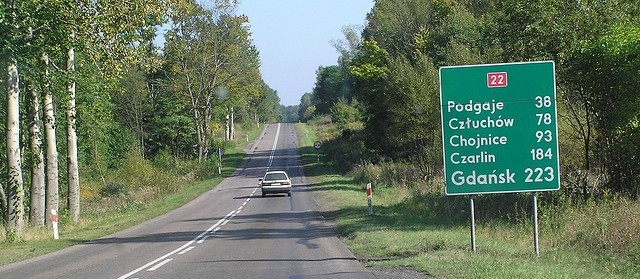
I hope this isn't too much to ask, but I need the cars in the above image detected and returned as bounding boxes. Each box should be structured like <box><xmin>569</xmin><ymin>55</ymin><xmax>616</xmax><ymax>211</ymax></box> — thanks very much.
<box><xmin>259</xmin><ymin>171</ymin><xmax>292</xmax><ymax>197</ymax></box>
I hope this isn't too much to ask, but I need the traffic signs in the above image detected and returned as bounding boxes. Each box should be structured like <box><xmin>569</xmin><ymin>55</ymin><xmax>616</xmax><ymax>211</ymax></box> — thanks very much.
<box><xmin>439</xmin><ymin>60</ymin><xmax>561</xmax><ymax>196</ymax></box>
